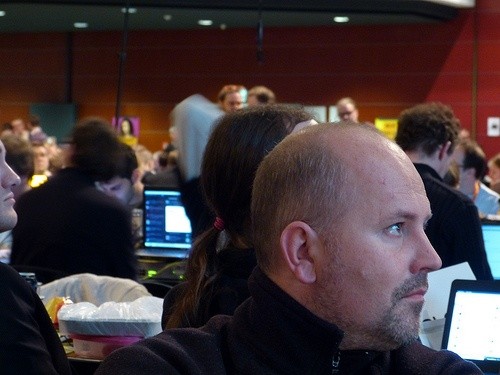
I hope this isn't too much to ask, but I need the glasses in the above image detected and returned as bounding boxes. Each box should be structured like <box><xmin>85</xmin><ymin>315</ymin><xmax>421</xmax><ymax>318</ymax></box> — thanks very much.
<box><xmin>338</xmin><ymin>110</ymin><xmax>356</xmax><ymax>118</ymax></box>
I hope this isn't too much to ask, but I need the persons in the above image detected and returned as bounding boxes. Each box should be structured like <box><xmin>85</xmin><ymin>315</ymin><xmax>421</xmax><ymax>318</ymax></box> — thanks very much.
<box><xmin>395</xmin><ymin>102</ymin><xmax>494</xmax><ymax>281</ymax></box>
<box><xmin>0</xmin><ymin>118</ymin><xmax>68</xmax><ymax>200</ymax></box>
<box><xmin>9</xmin><ymin>120</ymin><xmax>138</xmax><ymax>285</ymax></box>
<box><xmin>0</xmin><ymin>137</ymin><xmax>71</xmax><ymax>375</ymax></box>
<box><xmin>95</xmin><ymin>119</ymin><xmax>178</xmax><ymax>248</ymax></box>
<box><xmin>220</xmin><ymin>85</ymin><xmax>248</xmax><ymax>113</ymax></box>
<box><xmin>92</xmin><ymin>123</ymin><xmax>485</xmax><ymax>375</ymax></box>
<box><xmin>337</xmin><ymin>97</ymin><xmax>359</xmax><ymax>124</ymax></box>
<box><xmin>442</xmin><ymin>128</ymin><xmax>499</xmax><ymax>214</ymax></box>
<box><xmin>247</xmin><ymin>86</ymin><xmax>275</xmax><ymax>109</ymax></box>
<box><xmin>161</xmin><ymin>105</ymin><xmax>320</xmax><ymax>330</ymax></box>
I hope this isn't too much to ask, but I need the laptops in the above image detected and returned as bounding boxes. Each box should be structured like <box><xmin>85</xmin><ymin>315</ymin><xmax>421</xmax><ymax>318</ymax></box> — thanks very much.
<box><xmin>417</xmin><ymin>261</ymin><xmax>477</xmax><ymax>322</ymax></box>
<box><xmin>479</xmin><ymin>219</ymin><xmax>500</xmax><ymax>280</ymax></box>
<box><xmin>134</xmin><ymin>185</ymin><xmax>194</xmax><ymax>259</ymax></box>
<box><xmin>441</xmin><ymin>280</ymin><xmax>500</xmax><ymax>375</ymax></box>
